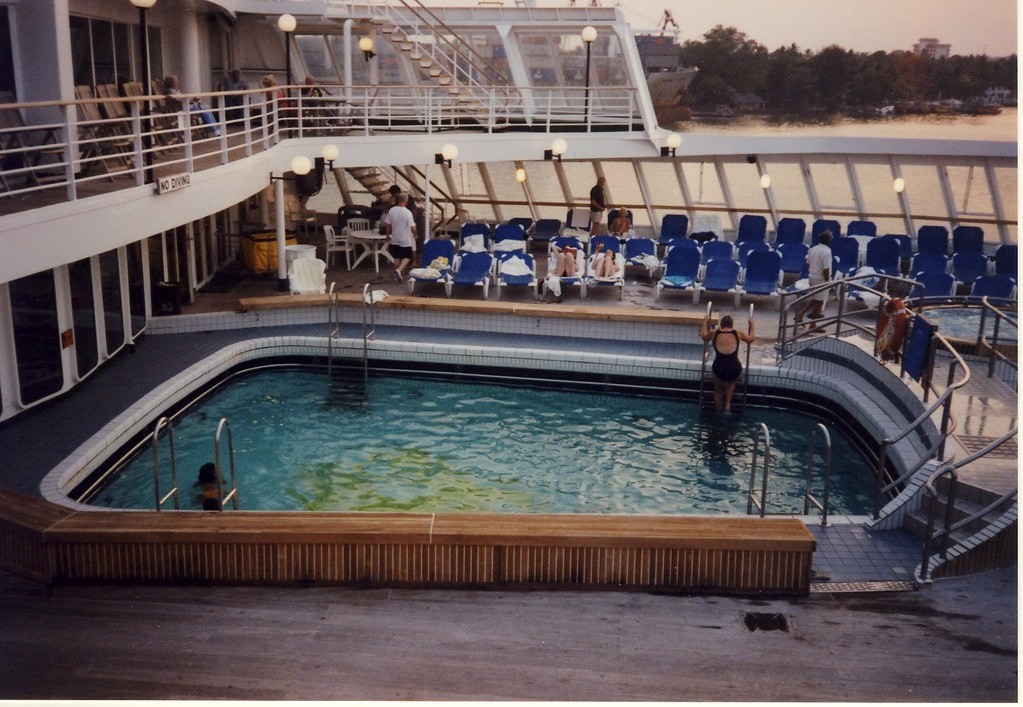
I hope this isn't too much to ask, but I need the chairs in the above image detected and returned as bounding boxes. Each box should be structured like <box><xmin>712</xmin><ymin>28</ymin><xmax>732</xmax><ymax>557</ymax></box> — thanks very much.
<box><xmin>287</xmin><ymin>200</ymin><xmax>320</xmax><ymax>238</ymax></box>
<box><xmin>349</xmin><ymin>218</ymin><xmax>376</xmax><ymax>262</ymax></box>
<box><xmin>0</xmin><ymin>89</ymin><xmax>66</xmax><ymax>194</ymax></box>
<box><xmin>75</xmin><ymin>78</ymin><xmax>208</xmax><ymax>182</ymax></box>
<box><xmin>407</xmin><ymin>210</ymin><xmax>1017</xmax><ymax>313</ymax></box>
<box><xmin>323</xmin><ymin>223</ymin><xmax>356</xmax><ymax>271</ymax></box>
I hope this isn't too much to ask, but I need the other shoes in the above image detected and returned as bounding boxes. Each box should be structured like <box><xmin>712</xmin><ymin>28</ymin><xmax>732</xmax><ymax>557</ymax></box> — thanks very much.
<box><xmin>794</xmin><ymin>317</ymin><xmax>805</xmax><ymax>327</ymax></box>
<box><xmin>809</xmin><ymin>328</ymin><xmax>825</xmax><ymax>333</ymax></box>
<box><xmin>394</xmin><ymin>270</ymin><xmax>403</xmax><ymax>282</ymax></box>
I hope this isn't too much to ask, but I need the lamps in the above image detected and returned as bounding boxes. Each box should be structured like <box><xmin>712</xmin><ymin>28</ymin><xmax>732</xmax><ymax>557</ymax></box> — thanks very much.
<box><xmin>359</xmin><ymin>37</ymin><xmax>376</xmax><ymax>62</ymax></box>
<box><xmin>435</xmin><ymin>142</ymin><xmax>460</xmax><ymax>169</ymax></box>
<box><xmin>324</xmin><ymin>146</ymin><xmax>340</xmax><ymax>172</ymax></box>
<box><xmin>544</xmin><ymin>138</ymin><xmax>570</xmax><ymax>163</ymax></box>
<box><xmin>660</xmin><ymin>132</ymin><xmax>684</xmax><ymax>158</ymax></box>
<box><xmin>269</xmin><ymin>154</ymin><xmax>314</xmax><ymax>184</ymax></box>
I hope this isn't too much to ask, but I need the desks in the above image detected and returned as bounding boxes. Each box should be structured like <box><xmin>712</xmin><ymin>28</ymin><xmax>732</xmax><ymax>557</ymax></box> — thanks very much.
<box><xmin>304</xmin><ymin>98</ymin><xmax>346</xmax><ymax>135</ymax></box>
<box><xmin>349</xmin><ymin>229</ymin><xmax>395</xmax><ymax>274</ymax></box>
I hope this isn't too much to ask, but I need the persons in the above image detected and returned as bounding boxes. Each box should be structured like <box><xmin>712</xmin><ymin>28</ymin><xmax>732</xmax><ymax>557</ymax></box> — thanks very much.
<box><xmin>701</xmin><ymin>314</ymin><xmax>755</xmax><ymax>409</ymax></box>
<box><xmin>610</xmin><ymin>207</ymin><xmax>631</xmax><ymax>237</ymax></box>
<box><xmin>263</xmin><ymin>74</ymin><xmax>313</xmax><ymax>133</ymax></box>
<box><xmin>388</xmin><ymin>185</ymin><xmax>420</xmax><ymax>267</ymax></box>
<box><xmin>793</xmin><ymin>230</ymin><xmax>834</xmax><ymax>333</ymax></box>
<box><xmin>592</xmin><ymin>242</ymin><xmax>619</xmax><ymax>278</ymax></box>
<box><xmin>589</xmin><ymin>177</ymin><xmax>606</xmax><ymax>235</ymax></box>
<box><xmin>217</xmin><ymin>70</ymin><xmax>247</xmax><ymax>119</ymax></box>
<box><xmin>303</xmin><ymin>76</ymin><xmax>335</xmax><ymax>110</ymax></box>
<box><xmin>385</xmin><ymin>193</ymin><xmax>418</xmax><ymax>282</ymax></box>
<box><xmin>553</xmin><ymin>241</ymin><xmax>578</xmax><ymax>276</ymax></box>
<box><xmin>197</xmin><ymin>462</ymin><xmax>216</xmax><ymax>483</ymax></box>
<box><xmin>164</xmin><ymin>75</ymin><xmax>219</xmax><ymax>135</ymax></box>
